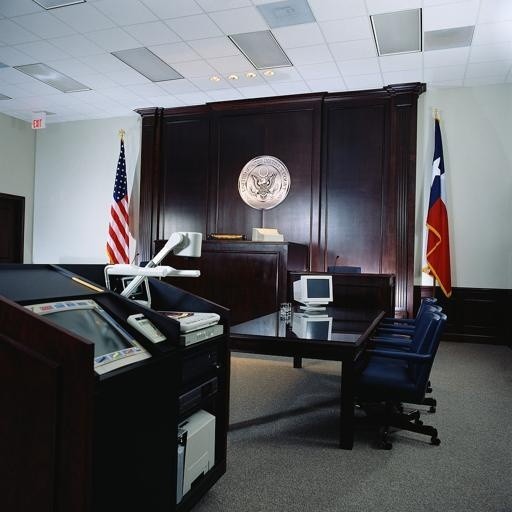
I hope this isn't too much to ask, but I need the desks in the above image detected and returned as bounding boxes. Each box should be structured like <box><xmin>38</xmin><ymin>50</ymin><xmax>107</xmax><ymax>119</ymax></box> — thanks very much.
<box><xmin>227</xmin><ymin>303</ymin><xmax>386</xmax><ymax>447</ymax></box>
<box><xmin>0</xmin><ymin>257</ymin><xmax>229</xmax><ymax>512</ymax></box>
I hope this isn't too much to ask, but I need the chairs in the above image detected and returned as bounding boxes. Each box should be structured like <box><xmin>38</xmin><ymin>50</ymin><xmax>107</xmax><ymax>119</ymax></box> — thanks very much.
<box><xmin>357</xmin><ymin>294</ymin><xmax>450</xmax><ymax>449</ymax></box>
<box><xmin>206</xmin><ymin>233</ymin><xmax>246</xmax><ymax>240</ymax></box>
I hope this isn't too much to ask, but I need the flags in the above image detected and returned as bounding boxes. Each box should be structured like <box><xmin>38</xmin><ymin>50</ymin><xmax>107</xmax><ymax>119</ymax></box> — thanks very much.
<box><xmin>106</xmin><ymin>130</ymin><xmax>129</xmax><ymax>264</ymax></box>
<box><xmin>421</xmin><ymin>107</ymin><xmax>452</xmax><ymax>298</ymax></box>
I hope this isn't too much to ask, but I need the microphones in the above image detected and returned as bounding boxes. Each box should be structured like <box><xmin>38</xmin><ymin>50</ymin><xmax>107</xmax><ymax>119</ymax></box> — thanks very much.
<box><xmin>334</xmin><ymin>255</ymin><xmax>339</xmax><ymax>272</ymax></box>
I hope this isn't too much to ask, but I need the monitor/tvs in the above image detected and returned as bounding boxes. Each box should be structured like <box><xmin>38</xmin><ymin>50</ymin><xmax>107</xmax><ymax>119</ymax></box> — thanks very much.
<box><xmin>23</xmin><ymin>298</ymin><xmax>154</xmax><ymax>377</ymax></box>
<box><xmin>252</xmin><ymin>227</ymin><xmax>284</xmax><ymax>242</ymax></box>
<box><xmin>291</xmin><ymin>312</ymin><xmax>332</xmax><ymax>341</ymax></box>
<box><xmin>293</xmin><ymin>274</ymin><xmax>334</xmax><ymax>311</ymax></box>
